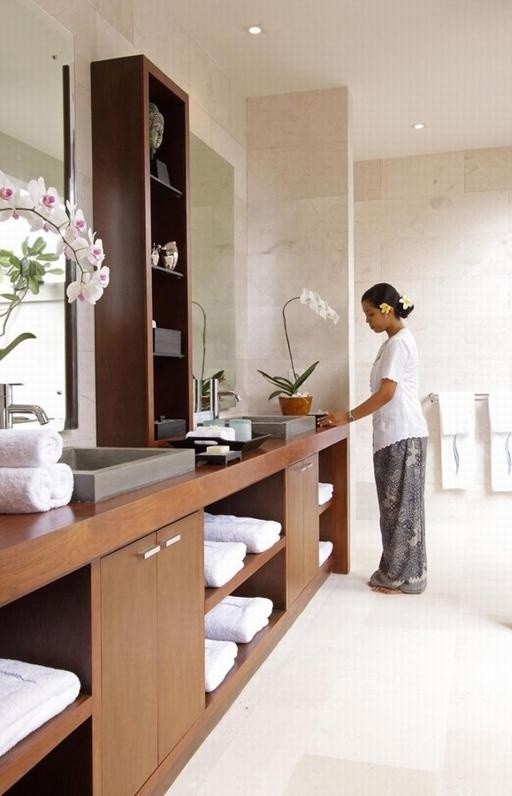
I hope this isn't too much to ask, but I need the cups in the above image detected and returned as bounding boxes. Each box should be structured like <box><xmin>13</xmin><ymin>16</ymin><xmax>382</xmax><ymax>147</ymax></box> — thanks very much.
<box><xmin>229</xmin><ymin>419</ymin><xmax>251</xmax><ymax>441</ymax></box>
<box><xmin>203</xmin><ymin>419</ymin><xmax>225</xmax><ymax>427</ymax></box>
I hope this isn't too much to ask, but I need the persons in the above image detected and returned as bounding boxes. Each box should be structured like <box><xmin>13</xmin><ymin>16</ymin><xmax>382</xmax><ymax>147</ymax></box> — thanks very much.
<box><xmin>316</xmin><ymin>283</ymin><xmax>429</xmax><ymax>594</ymax></box>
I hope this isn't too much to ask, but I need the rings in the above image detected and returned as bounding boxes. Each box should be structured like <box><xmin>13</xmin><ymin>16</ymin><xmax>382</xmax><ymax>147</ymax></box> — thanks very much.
<box><xmin>327</xmin><ymin>420</ymin><xmax>333</xmax><ymax>425</ymax></box>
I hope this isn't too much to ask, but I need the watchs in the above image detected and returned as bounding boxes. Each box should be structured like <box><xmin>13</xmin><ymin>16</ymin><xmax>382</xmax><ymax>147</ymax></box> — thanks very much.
<box><xmin>348</xmin><ymin>410</ymin><xmax>356</xmax><ymax>422</ymax></box>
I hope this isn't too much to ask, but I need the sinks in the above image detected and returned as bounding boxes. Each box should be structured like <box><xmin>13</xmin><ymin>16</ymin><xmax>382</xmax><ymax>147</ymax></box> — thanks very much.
<box><xmin>56</xmin><ymin>445</ymin><xmax>196</xmax><ymax>504</ymax></box>
<box><xmin>196</xmin><ymin>414</ymin><xmax>317</xmax><ymax>440</ymax></box>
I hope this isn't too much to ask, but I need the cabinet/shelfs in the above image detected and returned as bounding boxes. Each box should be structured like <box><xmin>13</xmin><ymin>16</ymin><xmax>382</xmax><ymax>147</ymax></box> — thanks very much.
<box><xmin>86</xmin><ymin>54</ymin><xmax>195</xmax><ymax>449</ymax></box>
<box><xmin>0</xmin><ymin>423</ymin><xmax>352</xmax><ymax>794</ymax></box>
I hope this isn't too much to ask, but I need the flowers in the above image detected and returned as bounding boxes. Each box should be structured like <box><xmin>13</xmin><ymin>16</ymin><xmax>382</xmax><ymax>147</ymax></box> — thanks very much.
<box><xmin>192</xmin><ymin>300</ymin><xmax>230</xmax><ymax>394</ymax></box>
<box><xmin>1</xmin><ymin>172</ymin><xmax>112</xmax><ymax>358</ymax></box>
<box><xmin>257</xmin><ymin>287</ymin><xmax>341</xmax><ymax>395</ymax></box>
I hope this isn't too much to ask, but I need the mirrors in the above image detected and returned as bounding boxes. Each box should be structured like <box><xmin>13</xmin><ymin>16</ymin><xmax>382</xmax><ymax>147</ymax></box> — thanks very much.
<box><xmin>0</xmin><ymin>1</ymin><xmax>81</xmax><ymax>435</ymax></box>
<box><xmin>190</xmin><ymin>130</ymin><xmax>241</xmax><ymax>414</ymax></box>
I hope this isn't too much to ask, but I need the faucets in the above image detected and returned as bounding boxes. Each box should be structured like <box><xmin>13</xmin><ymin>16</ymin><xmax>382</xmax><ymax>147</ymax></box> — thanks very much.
<box><xmin>192</xmin><ymin>378</ymin><xmax>202</xmax><ymax>413</ymax></box>
<box><xmin>0</xmin><ymin>383</ymin><xmax>49</xmax><ymax>429</ymax></box>
<box><xmin>209</xmin><ymin>377</ymin><xmax>242</xmax><ymax>420</ymax></box>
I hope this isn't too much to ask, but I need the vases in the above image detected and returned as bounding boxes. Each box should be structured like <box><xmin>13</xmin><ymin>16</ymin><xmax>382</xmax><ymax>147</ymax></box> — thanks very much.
<box><xmin>195</xmin><ymin>395</ymin><xmax>210</xmax><ymax>412</ymax></box>
<box><xmin>279</xmin><ymin>392</ymin><xmax>314</xmax><ymax>420</ymax></box>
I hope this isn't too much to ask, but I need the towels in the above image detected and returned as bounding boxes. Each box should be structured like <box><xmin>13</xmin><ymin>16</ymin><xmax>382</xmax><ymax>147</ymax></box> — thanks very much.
<box><xmin>185</xmin><ymin>425</ymin><xmax>235</xmax><ymax>441</ymax></box>
<box><xmin>318</xmin><ymin>534</ymin><xmax>335</xmax><ymax>564</ymax></box>
<box><xmin>202</xmin><ymin>511</ymin><xmax>283</xmax><ymax>554</ymax></box>
<box><xmin>316</xmin><ymin>477</ymin><xmax>337</xmax><ymax>507</ymax></box>
<box><xmin>4</xmin><ymin>463</ymin><xmax>73</xmax><ymax>517</ymax></box>
<box><xmin>3</xmin><ymin>431</ymin><xmax>66</xmax><ymax>467</ymax></box>
<box><xmin>436</xmin><ymin>389</ymin><xmax>474</xmax><ymax>490</ymax></box>
<box><xmin>205</xmin><ymin>634</ymin><xmax>239</xmax><ymax>690</ymax></box>
<box><xmin>202</xmin><ymin>538</ymin><xmax>246</xmax><ymax>590</ymax></box>
<box><xmin>206</xmin><ymin>593</ymin><xmax>272</xmax><ymax>647</ymax></box>
<box><xmin>484</xmin><ymin>390</ymin><xmax>512</xmax><ymax>493</ymax></box>
<box><xmin>0</xmin><ymin>657</ymin><xmax>80</xmax><ymax>755</ymax></box>
